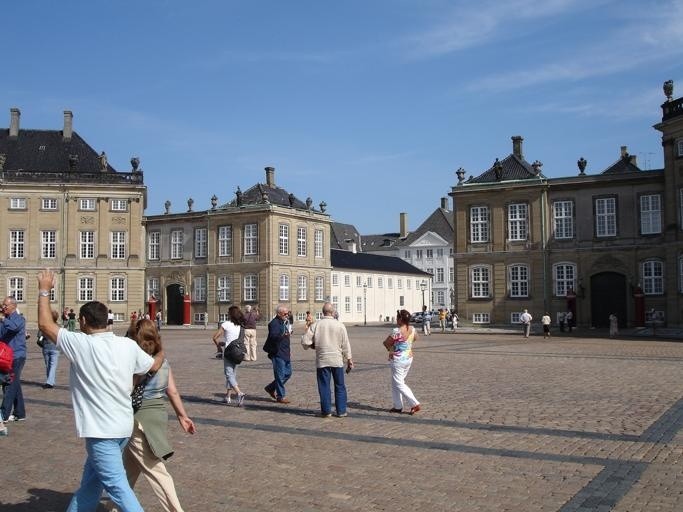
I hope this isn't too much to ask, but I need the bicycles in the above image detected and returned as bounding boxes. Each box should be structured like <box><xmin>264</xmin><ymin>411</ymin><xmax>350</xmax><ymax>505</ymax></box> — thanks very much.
<box><xmin>406</xmin><ymin>307</ymin><xmax>454</xmax><ymax>326</ymax></box>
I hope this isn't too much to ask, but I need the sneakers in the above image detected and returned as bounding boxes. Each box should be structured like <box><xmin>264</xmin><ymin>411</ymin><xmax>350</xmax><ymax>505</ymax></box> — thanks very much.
<box><xmin>237</xmin><ymin>392</ymin><xmax>245</xmax><ymax>406</ymax></box>
<box><xmin>224</xmin><ymin>396</ymin><xmax>231</xmax><ymax>403</ymax></box>
<box><xmin>410</xmin><ymin>406</ymin><xmax>420</xmax><ymax>415</ymax></box>
<box><xmin>264</xmin><ymin>386</ymin><xmax>290</xmax><ymax>403</ymax></box>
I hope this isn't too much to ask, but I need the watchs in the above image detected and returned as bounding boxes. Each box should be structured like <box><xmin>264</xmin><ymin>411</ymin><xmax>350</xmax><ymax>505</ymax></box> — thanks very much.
<box><xmin>38</xmin><ymin>289</ymin><xmax>52</xmax><ymax>298</ymax></box>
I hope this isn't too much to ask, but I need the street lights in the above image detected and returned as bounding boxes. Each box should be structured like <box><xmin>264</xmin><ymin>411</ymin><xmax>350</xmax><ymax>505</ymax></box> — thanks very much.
<box><xmin>449</xmin><ymin>286</ymin><xmax>453</xmax><ymax>310</ymax></box>
<box><xmin>215</xmin><ymin>285</ymin><xmax>222</xmax><ymax>329</ymax></box>
<box><xmin>420</xmin><ymin>278</ymin><xmax>426</xmax><ymax>305</ymax></box>
<box><xmin>361</xmin><ymin>282</ymin><xmax>367</xmax><ymax>326</ymax></box>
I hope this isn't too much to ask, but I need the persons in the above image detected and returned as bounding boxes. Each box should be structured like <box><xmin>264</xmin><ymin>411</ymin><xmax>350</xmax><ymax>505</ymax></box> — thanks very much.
<box><xmin>107</xmin><ymin>309</ymin><xmax>163</xmax><ymax>341</ymax></box>
<box><xmin>455</xmin><ymin>157</ymin><xmax>587</xmax><ymax>182</ymax></box>
<box><xmin>1</xmin><ymin>296</ymin><xmax>77</xmax><ymax>435</ymax></box>
<box><xmin>383</xmin><ymin>306</ymin><xmax>459</xmax><ymax>415</ymax></box>
<box><xmin>164</xmin><ymin>185</ymin><xmax>328</xmax><ymax>214</ymax></box>
<box><xmin>95</xmin><ymin>151</ymin><xmax>108</xmax><ymax>173</ymax></box>
<box><xmin>520</xmin><ymin>309</ymin><xmax>619</xmax><ymax>339</ymax></box>
<box><xmin>98</xmin><ymin>314</ymin><xmax>198</xmax><ymax>512</ymax></box>
<box><xmin>203</xmin><ymin>302</ymin><xmax>353</xmax><ymax>418</ymax></box>
<box><xmin>33</xmin><ymin>262</ymin><xmax>166</xmax><ymax>512</ymax></box>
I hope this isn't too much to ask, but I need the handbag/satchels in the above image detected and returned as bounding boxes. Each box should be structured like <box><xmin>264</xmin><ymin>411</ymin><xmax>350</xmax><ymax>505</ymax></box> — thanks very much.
<box><xmin>224</xmin><ymin>339</ymin><xmax>247</xmax><ymax>364</ymax></box>
<box><xmin>0</xmin><ymin>342</ymin><xmax>14</xmax><ymax>372</ymax></box>
